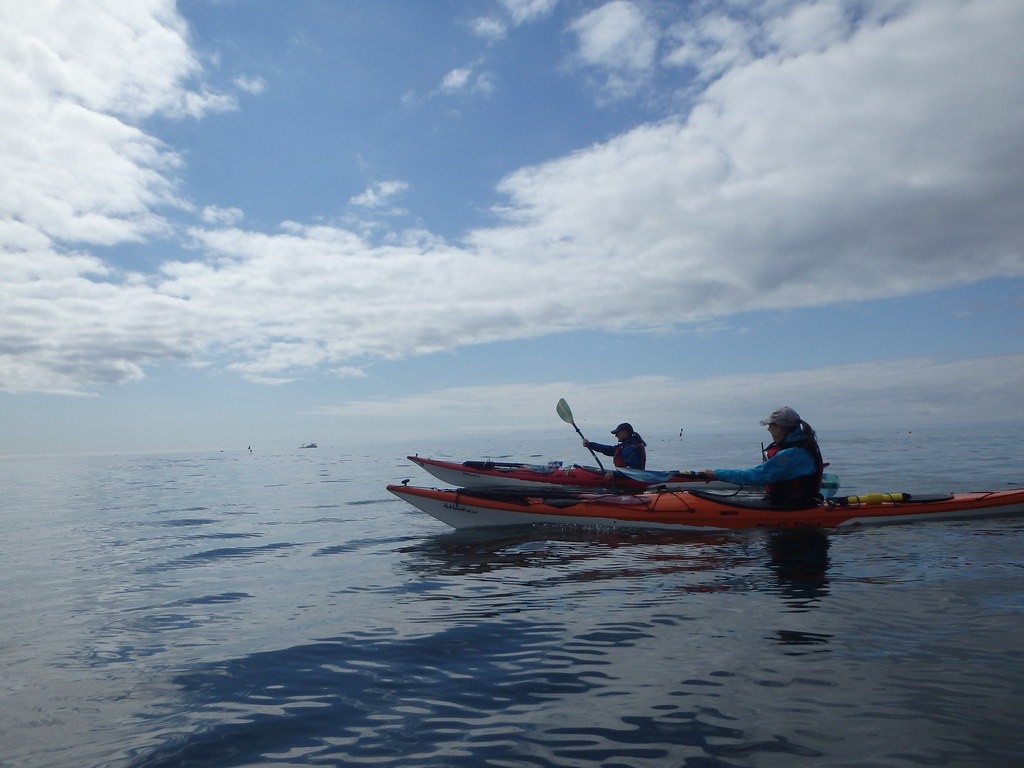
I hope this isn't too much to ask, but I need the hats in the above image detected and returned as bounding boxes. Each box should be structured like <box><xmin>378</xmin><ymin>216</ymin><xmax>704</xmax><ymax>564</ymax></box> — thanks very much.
<box><xmin>760</xmin><ymin>406</ymin><xmax>800</xmax><ymax>427</ymax></box>
<box><xmin>611</xmin><ymin>423</ymin><xmax>633</xmax><ymax>434</ymax></box>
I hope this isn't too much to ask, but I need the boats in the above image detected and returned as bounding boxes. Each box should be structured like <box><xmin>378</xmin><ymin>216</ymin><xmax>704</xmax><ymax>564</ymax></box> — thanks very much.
<box><xmin>405</xmin><ymin>454</ymin><xmax>770</xmax><ymax>507</ymax></box>
<box><xmin>384</xmin><ymin>479</ymin><xmax>1024</xmax><ymax>535</ymax></box>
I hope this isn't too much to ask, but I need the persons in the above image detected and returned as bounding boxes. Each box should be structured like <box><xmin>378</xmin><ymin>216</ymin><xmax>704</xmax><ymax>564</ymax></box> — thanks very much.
<box><xmin>583</xmin><ymin>423</ymin><xmax>646</xmax><ymax>469</ymax></box>
<box><xmin>703</xmin><ymin>406</ymin><xmax>824</xmax><ymax>508</ymax></box>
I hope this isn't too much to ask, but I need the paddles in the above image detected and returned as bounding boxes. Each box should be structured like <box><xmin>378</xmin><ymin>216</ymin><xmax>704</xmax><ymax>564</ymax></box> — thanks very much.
<box><xmin>556</xmin><ymin>398</ymin><xmax>605</xmax><ymax>471</ymax></box>
<box><xmin>617</xmin><ymin>466</ymin><xmax>841</xmax><ymax>498</ymax></box>
